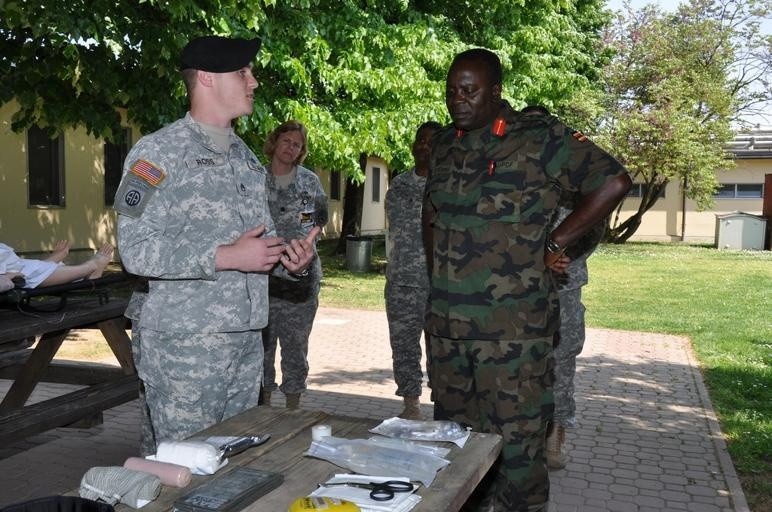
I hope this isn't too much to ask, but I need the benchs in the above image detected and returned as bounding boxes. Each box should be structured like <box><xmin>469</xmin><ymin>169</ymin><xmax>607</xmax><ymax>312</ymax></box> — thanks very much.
<box><xmin>0</xmin><ymin>373</ymin><xmax>145</xmax><ymax>448</ymax></box>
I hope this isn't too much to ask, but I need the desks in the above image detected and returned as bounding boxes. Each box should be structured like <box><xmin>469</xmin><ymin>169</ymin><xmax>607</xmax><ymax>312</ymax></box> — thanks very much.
<box><xmin>0</xmin><ymin>291</ymin><xmax>144</xmax><ymax>452</ymax></box>
<box><xmin>65</xmin><ymin>403</ymin><xmax>505</xmax><ymax>512</ymax></box>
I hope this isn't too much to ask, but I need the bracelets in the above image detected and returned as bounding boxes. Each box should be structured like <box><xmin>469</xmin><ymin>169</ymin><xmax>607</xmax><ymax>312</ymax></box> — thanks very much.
<box><xmin>295</xmin><ymin>264</ymin><xmax>314</xmax><ymax>280</ymax></box>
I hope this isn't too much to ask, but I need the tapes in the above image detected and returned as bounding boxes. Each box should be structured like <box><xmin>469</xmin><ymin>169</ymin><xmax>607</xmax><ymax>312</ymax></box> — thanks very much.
<box><xmin>311</xmin><ymin>424</ymin><xmax>332</xmax><ymax>441</ymax></box>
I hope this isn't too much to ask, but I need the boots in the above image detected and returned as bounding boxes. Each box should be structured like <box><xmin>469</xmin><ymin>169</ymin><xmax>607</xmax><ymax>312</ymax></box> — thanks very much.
<box><xmin>258</xmin><ymin>389</ymin><xmax>271</xmax><ymax>406</ymax></box>
<box><xmin>285</xmin><ymin>392</ymin><xmax>301</xmax><ymax>409</ymax></box>
<box><xmin>396</xmin><ymin>395</ymin><xmax>423</xmax><ymax>420</ymax></box>
<box><xmin>543</xmin><ymin>417</ymin><xmax>568</xmax><ymax>470</ymax></box>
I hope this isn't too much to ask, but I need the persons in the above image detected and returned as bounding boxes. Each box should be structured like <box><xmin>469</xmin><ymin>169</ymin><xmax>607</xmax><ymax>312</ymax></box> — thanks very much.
<box><xmin>259</xmin><ymin>120</ymin><xmax>328</xmax><ymax>412</ymax></box>
<box><xmin>384</xmin><ymin>121</ymin><xmax>444</xmax><ymax>420</ymax></box>
<box><xmin>113</xmin><ymin>35</ymin><xmax>322</xmax><ymax>461</ymax></box>
<box><xmin>0</xmin><ymin>239</ymin><xmax>113</xmax><ymax>294</ymax></box>
<box><xmin>421</xmin><ymin>48</ymin><xmax>633</xmax><ymax>512</ymax></box>
<box><xmin>520</xmin><ymin>106</ymin><xmax>607</xmax><ymax>469</ymax></box>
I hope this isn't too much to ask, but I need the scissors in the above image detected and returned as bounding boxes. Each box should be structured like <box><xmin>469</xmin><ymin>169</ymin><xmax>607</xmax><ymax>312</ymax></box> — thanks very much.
<box><xmin>317</xmin><ymin>480</ymin><xmax>413</xmax><ymax>501</ymax></box>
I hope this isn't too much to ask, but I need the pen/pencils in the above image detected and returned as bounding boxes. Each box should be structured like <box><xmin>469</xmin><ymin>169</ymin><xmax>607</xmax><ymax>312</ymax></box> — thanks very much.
<box><xmin>489</xmin><ymin>158</ymin><xmax>495</xmax><ymax>176</ymax></box>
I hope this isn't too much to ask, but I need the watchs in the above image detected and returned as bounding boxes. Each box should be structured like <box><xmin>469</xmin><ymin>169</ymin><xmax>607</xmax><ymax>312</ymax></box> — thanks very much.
<box><xmin>546</xmin><ymin>239</ymin><xmax>560</xmax><ymax>255</ymax></box>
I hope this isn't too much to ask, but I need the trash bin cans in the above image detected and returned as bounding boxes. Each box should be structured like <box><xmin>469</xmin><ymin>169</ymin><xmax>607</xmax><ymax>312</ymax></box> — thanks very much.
<box><xmin>346</xmin><ymin>235</ymin><xmax>373</xmax><ymax>273</ymax></box>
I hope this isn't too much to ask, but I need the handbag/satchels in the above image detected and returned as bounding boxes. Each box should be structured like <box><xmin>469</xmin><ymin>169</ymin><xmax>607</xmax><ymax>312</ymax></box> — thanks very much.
<box><xmin>268</xmin><ymin>274</ymin><xmax>312</xmax><ymax>305</ymax></box>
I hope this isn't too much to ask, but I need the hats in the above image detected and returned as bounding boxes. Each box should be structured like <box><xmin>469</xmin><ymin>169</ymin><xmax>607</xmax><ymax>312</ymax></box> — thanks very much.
<box><xmin>179</xmin><ymin>35</ymin><xmax>262</xmax><ymax>74</ymax></box>
